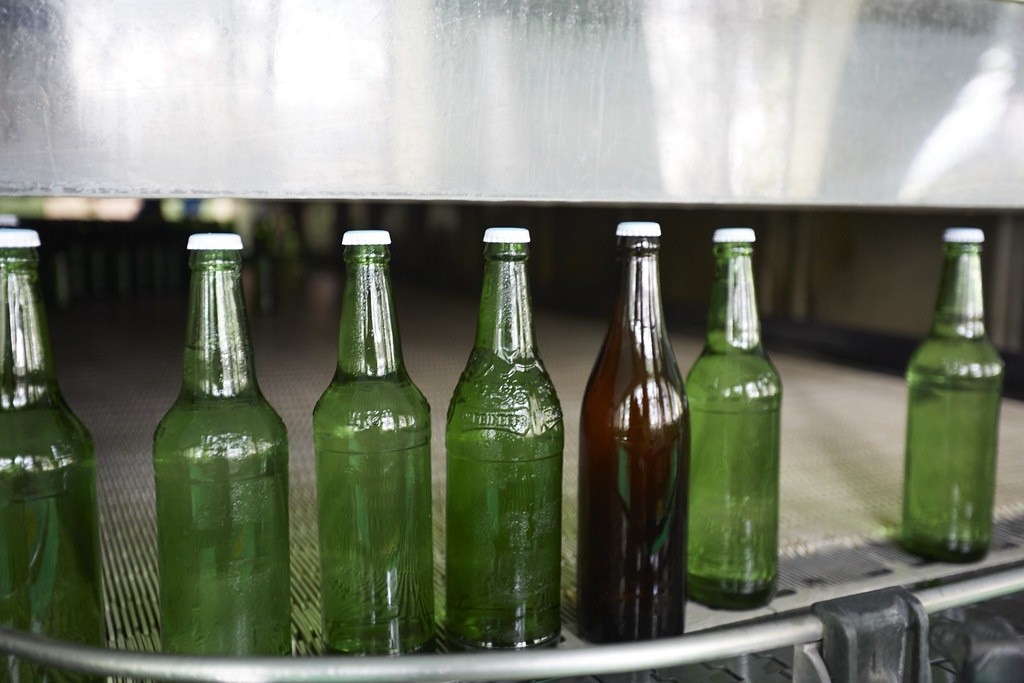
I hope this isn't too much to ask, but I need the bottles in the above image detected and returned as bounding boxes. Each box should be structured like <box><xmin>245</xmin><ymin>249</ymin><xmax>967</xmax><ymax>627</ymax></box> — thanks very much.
<box><xmin>446</xmin><ymin>227</ymin><xmax>564</xmax><ymax>653</ymax></box>
<box><xmin>153</xmin><ymin>233</ymin><xmax>291</xmax><ymax>662</ymax></box>
<box><xmin>0</xmin><ymin>213</ymin><xmax>110</xmax><ymax>683</ymax></box>
<box><xmin>902</xmin><ymin>228</ymin><xmax>1006</xmax><ymax>563</ymax></box>
<box><xmin>574</xmin><ymin>221</ymin><xmax>691</xmax><ymax>644</ymax></box>
<box><xmin>684</xmin><ymin>229</ymin><xmax>782</xmax><ymax>612</ymax></box>
<box><xmin>312</xmin><ymin>229</ymin><xmax>430</xmax><ymax>656</ymax></box>
